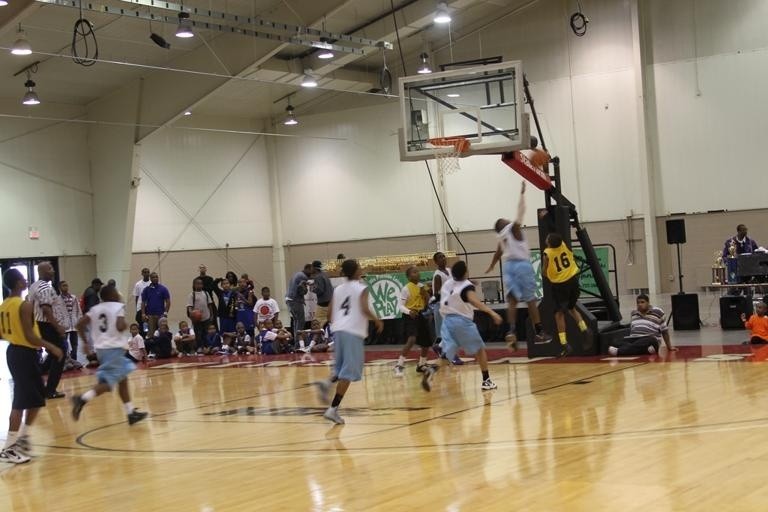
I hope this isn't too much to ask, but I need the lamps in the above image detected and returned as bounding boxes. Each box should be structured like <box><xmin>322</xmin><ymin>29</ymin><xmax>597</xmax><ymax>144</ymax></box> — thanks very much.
<box><xmin>317</xmin><ymin>37</ymin><xmax>334</xmax><ymax>58</ymax></box>
<box><xmin>433</xmin><ymin>2</ymin><xmax>461</xmax><ymax>23</ymax></box>
<box><xmin>283</xmin><ymin>96</ymin><xmax>298</xmax><ymax>125</ymax></box>
<box><xmin>446</xmin><ymin>23</ymin><xmax>461</xmax><ymax>98</ymax></box>
<box><xmin>416</xmin><ymin>53</ymin><xmax>432</xmax><ymax>74</ymax></box>
<box><xmin>300</xmin><ymin>67</ymin><xmax>322</xmax><ymax>88</ymax></box>
<box><xmin>175</xmin><ymin>12</ymin><xmax>194</xmax><ymax>38</ymax></box>
<box><xmin>22</xmin><ymin>70</ymin><xmax>40</xmax><ymax>105</ymax></box>
<box><xmin>11</xmin><ymin>23</ymin><xmax>32</xmax><ymax>55</ymax></box>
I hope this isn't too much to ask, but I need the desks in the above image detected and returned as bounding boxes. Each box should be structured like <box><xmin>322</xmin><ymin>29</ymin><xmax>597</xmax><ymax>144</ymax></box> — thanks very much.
<box><xmin>701</xmin><ymin>283</ymin><xmax>768</xmax><ymax>324</ymax></box>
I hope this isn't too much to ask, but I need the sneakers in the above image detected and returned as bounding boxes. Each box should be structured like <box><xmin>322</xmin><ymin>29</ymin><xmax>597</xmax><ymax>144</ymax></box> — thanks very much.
<box><xmin>322</xmin><ymin>408</ymin><xmax>345</xmax><ymax>424</ymax></box>
<box><xmin>0</xmin><ymin>391</ymin><xmax>147</xmax><ymax>463</ymax></box>
<box><xmin>317</xmin><ymin>380</ymin><xmax>331</xmax><ymax>405</ymax></box>
<box><xmin>297</xmin><ymin>345</ymin><xmax>312</xmax><ymax>352</ymax></box>
<box><xmin>393</xmin><ymin>331</ymin><xmax>655</xmax><ymax>391</ymax></box>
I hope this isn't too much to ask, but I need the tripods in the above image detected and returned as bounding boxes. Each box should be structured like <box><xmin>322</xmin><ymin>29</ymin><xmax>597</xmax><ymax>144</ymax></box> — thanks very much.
<box><xmin>665</xmin><ymin>244</ymin><xmax>704</xmax><ymax>328</ymax></box>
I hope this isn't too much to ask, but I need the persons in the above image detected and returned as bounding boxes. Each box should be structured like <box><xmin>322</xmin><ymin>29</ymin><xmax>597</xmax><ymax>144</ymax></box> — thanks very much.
<box><xmin>108</xmin><ymin>279</ymin><xmax>120</xmax><ymax>294</ymax></box>
<box><xmin>149</xmin><ymin>316</ymin><xmax>335</xmax><ymax>360</ymax></box>
<box><xmin>133</xmin><ymin>268</ymin><xmax>171</xmax><ymax>340</ymax></box>
<box><xmin>423</xmin><ymin>261</ymin><xmax>502</xmax><ymax>392</ymax></box>
<box><xmin>29</xmin><ymin>262</ymin><xmax>69</xmax><ymax>399</ymax></box>
<box><xmin>2</xmin><ymin>269</ymin><xmax>64</xmax><ymax>464</ymax></box>
<box><xmin>126</xmin><ymin>323</ymin><xmax>147</xmax><ymax>366</ymax></box>
<box><xmin>318</xmin><ymin>260</ymin><xmax>384</xmax><ymax>424</ymax></box>
<box><xmin>59</xmin><ymin>280</ymin><xmax>80</xmax><ymax>370</ymax></box>
<box><xmin>186</xmin><ymin>264</ymin><xmax>258</xmax><ymax>351</ymax></box>
<box><xmin>541</xmin><ymin>223</ymin><xmax>594</xmax><ymax>360</ymax></box>
<box><xmin>82</xmin><ymin>279</ymin><xmax>103</xmax><ymax>366</ymax></box>
<box><xmin>741</xmin><ymin>302</ymin><xmax>768</xmax><ymax>344</ymax></box>
<box><xmin>483</xmin><ymin>178</ymin><xmax>551</xmax><ymax>343</ymax></box>
<box><xmin>309</xmin><ymin>260</ymin><xmax>333</xmax><ymax>329</ymax></box>
<box><xmin>722</xmin><ymin>223</ymin><xmax>761</xmax><ymax>296</ymax></box>
<box><xmin>285</xmin><ymin>264</ymin><xmax>312</xmax><ymax>341</ymax></box>
<box><xmin>253</xmin><ymin>287</ymin><xmax>280</xmax><ymax>323</ymax></box>
<box><xmin>71</xmin><ymin>285</ymin><xmax>147</xmax><ymax>424</ymax></box>
<box><xmin>430</xmin><ymin>253</ymin><xmax>463</xmax><ymax>365</ymax></box>
<box><xmin>606</xmin><ymin>293</ymin><xmax>680</xmax><ymax>356</ymax></box>
<box><xmin>395</xmin><ymin>267</ymin><xmax>433</xmax><ymax>373</ymax></box>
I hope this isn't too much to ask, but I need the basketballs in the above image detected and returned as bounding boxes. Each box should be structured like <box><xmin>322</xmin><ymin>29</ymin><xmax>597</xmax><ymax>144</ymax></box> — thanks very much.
<box><xmin>525</xmin><ymin>148</ymin><xmax>547</xmax><ymax>167</ymax></box>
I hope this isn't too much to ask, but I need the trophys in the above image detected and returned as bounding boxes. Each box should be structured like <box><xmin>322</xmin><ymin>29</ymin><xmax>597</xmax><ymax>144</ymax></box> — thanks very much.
<box><xmin>712</xmin><ymin>248</ymin><xmax>728</xmax><ymax>268</ymax></box>
<box><xmin>727</xmin><ymin>241</ymin><xmax>737</xmax><ymax>258</ymax></box>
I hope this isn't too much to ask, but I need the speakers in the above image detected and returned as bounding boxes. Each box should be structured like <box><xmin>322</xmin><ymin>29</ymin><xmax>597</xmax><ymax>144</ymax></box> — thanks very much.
<box><xmin>666</xmin><ymin>219</ymin><xmax>686</xmax><ymax>243</ymax></box>
<box><xmin>671</xmin><ymin>293</ymin><xmax>701</xmax><ymax>330</ymax></box>
<box><xmin>720</xmin><ymin>294</ymin><xmax>753</xmax><ymax>330</ymax></box>
